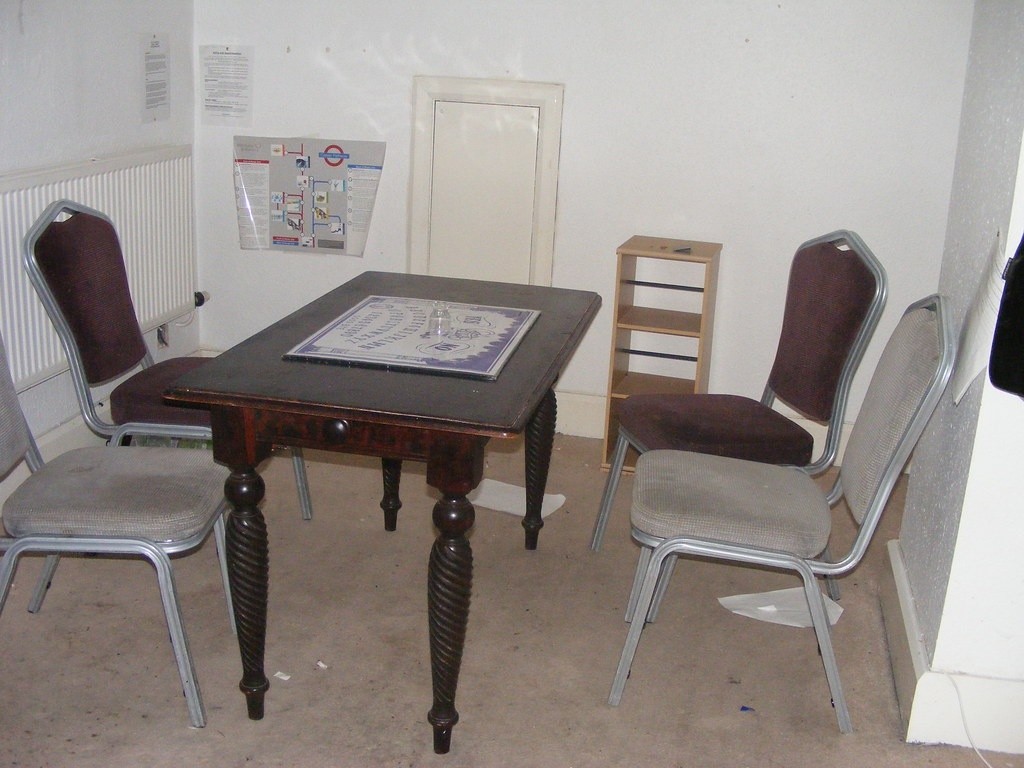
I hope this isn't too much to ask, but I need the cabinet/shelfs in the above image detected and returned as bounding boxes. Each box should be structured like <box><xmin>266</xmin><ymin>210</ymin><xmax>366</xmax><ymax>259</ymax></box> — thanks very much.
<box><xmin>600</xmin><ymin>235</ymin><xmax>724</xmax><ymax>470</ymax></box>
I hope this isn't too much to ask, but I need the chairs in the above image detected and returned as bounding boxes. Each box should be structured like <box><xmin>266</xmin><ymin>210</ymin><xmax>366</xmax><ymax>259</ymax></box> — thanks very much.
<box><xmin>587</xmin><ymin>228</ymin><xmax>887</xmax><ymax>624</ymax></box>
<box><xmin>21</xmin><ymin>194</ymin><xmax>314</xmax><ymax>540</ymax></box>
<box><xmin>0</xmin><ymin>338</ymin><xmax>234</xmax><ymax>728</ymax></box>
<box><xmin>612</xmin><ymin>291</ymin><xmax>961</xmax><ymax>733</ymax></box>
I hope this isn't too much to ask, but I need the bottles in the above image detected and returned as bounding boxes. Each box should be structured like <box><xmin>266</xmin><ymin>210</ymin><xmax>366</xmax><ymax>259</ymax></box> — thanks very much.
<box><xmin>428</xmin><ymin>301</ymin><xmax>452</xmax><ymax>335</ymax></box>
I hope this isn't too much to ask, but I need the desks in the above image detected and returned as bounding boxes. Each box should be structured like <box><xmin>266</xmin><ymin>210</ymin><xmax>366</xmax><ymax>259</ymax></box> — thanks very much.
<box><xmin>162</xmin><ymin>272</ymin><xmax>604</xmax><ymax>755</ymax></box>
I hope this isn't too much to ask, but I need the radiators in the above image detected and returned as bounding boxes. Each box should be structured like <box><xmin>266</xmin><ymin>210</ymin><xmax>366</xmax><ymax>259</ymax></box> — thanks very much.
<box><xmin>0</xmin><ymin>144</ymin><xmax>209</xmax><ymax>396</ymax></box>
